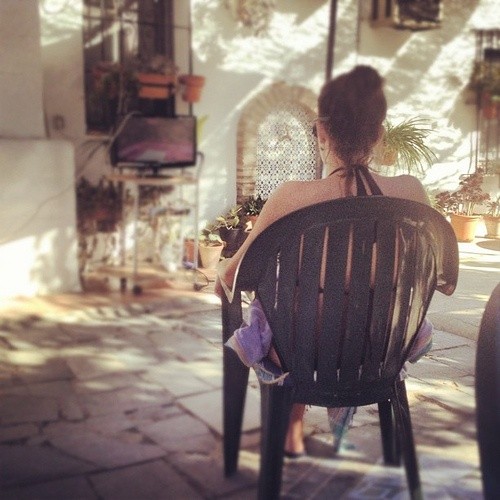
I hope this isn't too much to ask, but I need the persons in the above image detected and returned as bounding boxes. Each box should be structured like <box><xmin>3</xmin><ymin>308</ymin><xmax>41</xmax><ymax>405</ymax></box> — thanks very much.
<box><xmin>214</xmin><ymin>65</ymin><xmax>432</xmax><ymax>459</ymax></box>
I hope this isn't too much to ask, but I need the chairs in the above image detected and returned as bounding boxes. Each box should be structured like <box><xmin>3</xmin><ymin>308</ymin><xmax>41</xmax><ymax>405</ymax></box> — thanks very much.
<box><xmin>216</xmin><ymin>195</ymin><xmax>459</xmax><ymax>500</ymax></box>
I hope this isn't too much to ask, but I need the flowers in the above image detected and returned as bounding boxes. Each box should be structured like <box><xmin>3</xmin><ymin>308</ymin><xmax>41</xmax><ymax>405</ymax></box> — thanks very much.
<box><xmin>90</xmin><ymin>54</ymin><xmax>180</xmax><ymax>93</ymax></box>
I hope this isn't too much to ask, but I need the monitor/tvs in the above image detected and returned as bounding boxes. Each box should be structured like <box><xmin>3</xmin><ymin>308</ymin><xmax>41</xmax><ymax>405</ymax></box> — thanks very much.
<box><xmin>111</xmin><ymin>112</ymin><xmax>197</xmax><ymax>178</ymax></box>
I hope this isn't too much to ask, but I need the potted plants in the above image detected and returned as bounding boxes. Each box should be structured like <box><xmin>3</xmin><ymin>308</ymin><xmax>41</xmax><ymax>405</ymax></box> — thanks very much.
<box><xmin>238</xmin><ymin>194</ymin><xmax>268</xmax><ymax>231</ymax></box>
<box><xmin>434</xmin><ymin>166</ymin><xmax>490</xmax><ymax>242</ymax></box>
<box><xmin>217</xmin><ymin>206</ymin><xmax>241</xmax><ymax>250</ymax></box>
<box><xmin>78</xmin><ymin>175</ymin><xmax>113</xmax><ymax>221</ymax></box>
<box><xmin>199</xmin><ymin>228</ymin><xmax>225</xmax><ymax>268</ymax></box>
<box><xmin>374</xmin><ymin>114</ymin><xmax>440</xmax><ymax>181</ymax></box>
<box><xmin>185</xmin><ymin>238</ymin><xmax>196</xmax><ymax>262</ymax></box>
<box><xmin>468</xmin><ymin>58</ymin><xmax>500</xmax><ymax>119</ymax></box>
<box><xmin>483</xmin><ymin>196</ymin><xmax>500</xmax><ymax>238</ymax></box>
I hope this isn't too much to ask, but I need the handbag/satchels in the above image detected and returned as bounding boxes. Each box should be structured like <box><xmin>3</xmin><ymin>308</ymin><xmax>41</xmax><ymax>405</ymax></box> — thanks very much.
<box><xmin>109</xmin><ymin>108</ymin><xmax>198</xmax><ymax>169</ymax></box>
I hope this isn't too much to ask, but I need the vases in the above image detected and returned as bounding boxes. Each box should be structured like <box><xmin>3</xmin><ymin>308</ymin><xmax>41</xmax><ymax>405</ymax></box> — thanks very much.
<box><xmin>136</xmin><ymin>73</ymin><xmax>173</xmax><ymax>98</ymax></box>
<box><xmin>177</xmin><ymin>75</ymin><xmax>204</xmax><ymax>102</ymax></box>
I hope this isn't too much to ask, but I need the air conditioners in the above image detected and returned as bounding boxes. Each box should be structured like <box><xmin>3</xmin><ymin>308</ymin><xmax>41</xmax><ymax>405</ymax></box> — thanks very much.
<box><xmin>368</xmin><ymin>0</ymin><xmax>444</xmax><ymax>32</ymax></box>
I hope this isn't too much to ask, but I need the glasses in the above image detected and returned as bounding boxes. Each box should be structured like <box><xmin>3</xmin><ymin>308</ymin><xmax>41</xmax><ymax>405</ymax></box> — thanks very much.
<box><xmin>312</xmin><ymin>117</ymin><xmax>329</xmax><ymax>136</ymax></box>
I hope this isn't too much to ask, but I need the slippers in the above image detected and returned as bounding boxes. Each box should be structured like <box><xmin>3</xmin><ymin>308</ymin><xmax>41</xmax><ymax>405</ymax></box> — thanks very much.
<box><xmin>283</xmin><ymin>444</ymin><xmax>308</xmax><ymax>459</ymax></box>
